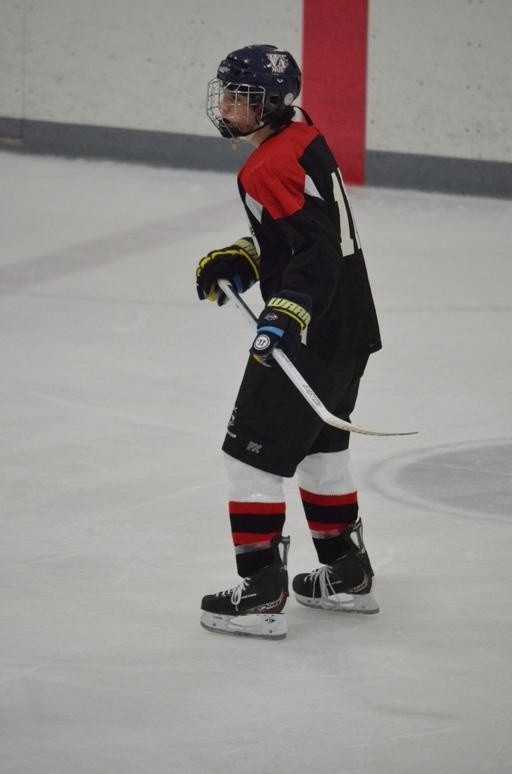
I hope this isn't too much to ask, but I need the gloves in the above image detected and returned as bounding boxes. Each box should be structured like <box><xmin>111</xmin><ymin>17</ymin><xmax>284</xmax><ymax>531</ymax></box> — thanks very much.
<box><xmin>196</xmin><ymin>237</ymin><xmax>261</xmax><ymax>306</ymax></box>
<box><xmin>249</xmin><ymin>297</ymin><xmax>312</xmax><ymax>368</ymax></box>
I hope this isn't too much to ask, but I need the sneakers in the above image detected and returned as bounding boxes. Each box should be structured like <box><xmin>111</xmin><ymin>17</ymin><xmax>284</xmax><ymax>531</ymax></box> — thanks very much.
<box><xmin>202</xmin><ymin>562</ymin><xmax>287</xmax><ymax>615</ymax></box>
<box><xmin>293</xmin><ymin>549</ymin><xmax>372</xmax><ymax>600</ymax></box>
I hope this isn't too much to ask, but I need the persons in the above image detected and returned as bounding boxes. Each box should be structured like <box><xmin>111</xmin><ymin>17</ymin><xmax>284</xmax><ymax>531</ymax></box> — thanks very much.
<box><xmin>198</xmin><ymin>44</ymin><xmax>383</xmax><ymax>636</ymax></box>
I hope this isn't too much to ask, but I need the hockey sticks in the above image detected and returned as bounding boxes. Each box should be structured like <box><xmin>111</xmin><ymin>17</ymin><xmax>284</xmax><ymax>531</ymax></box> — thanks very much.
<box><xmin>219</xmin><ymin>279</ymin><xmax>417</xmax><ymax>435</ymax></box>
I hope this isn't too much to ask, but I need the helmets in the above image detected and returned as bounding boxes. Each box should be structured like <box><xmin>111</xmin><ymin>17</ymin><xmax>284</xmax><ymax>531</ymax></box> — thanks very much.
<box><xmin>206</xmin><ymin>45</ymin><xmax>301</xmax><ymax>138</ymax></box>
